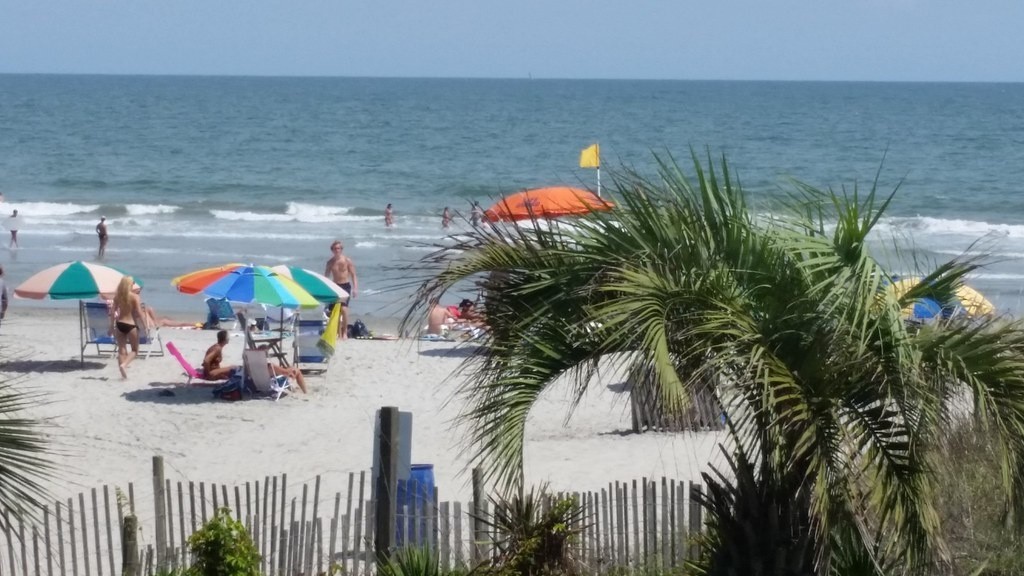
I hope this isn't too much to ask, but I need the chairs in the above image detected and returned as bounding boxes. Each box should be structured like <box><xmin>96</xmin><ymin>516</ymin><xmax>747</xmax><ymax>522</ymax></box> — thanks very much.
<box><xmin>238</xmin><ymin>312</ymin><xmax>290</xmax><ymax>367</ymax></box>
<box><xmin>290</xmin><ymin>317</ymin><xmax>332</xmax><ymax>374</ymax></box>
<box><xmin>206</xmin><ymin>298</ymin><xmax>242</xmax><ymax>331</ymax></box>
<box><xmin>78</xmin><ymin>301</ymin><xmax>164</xmax><ymax>360</ymax></box>
<box><xmin>166</xmin><ymin>342</ymin><xmax>226</xmax><ymax>390</ymax></box>
<box><xmin>242</xmin><ymin>348</ymin><xmax>294</xmax><ymax>402</ymax></box>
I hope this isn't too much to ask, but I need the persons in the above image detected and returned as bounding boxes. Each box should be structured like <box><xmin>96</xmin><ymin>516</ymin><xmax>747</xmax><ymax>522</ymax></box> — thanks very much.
<box><xmin>0</xmin><ymin>265</ymin><xmax>9</xmax><ymax>325</ymax></box>
<box><xmin>471</xmin><ymin>201</ymin><xmax>480</xmax><ymax>225</ymax></box>
<box><xmin>95</xmin><ymin>216</ymin><xmax>108</xmax><ymax>255</ymax></box>
<box><xmin>385</xmin><ymin>204</ymin><xmax>393</xmax><ymax>226</ymax></box>
<box><xmin>442</xmin><ymin>207</ymin><xmax>449</xmax><ymax>228</ymax></box>
<box><xmin>202</xmin><ymin>331</ymin><xmax>235</xmax><ymax>380</ymax></box>
<box><xmin>9</xmin><ymin>209</ymin><xmax>19</xmax><ymax>248</ymax></box>
<box><xmin>325</xmin><ymin>241</ymin><xmax>358</xmax><ymax>340</ymax></box>
<box><xmin>107</xmin><ymin>275</ymin><xmax>156</xmax><ymax>378</ymax></box>
<box><xmin>427</xmin><ymin>297</ymin><xmax>484</xmax><ymax>334</ymax></box>
<box><xmin>161</xmin><ymin>319</ymin><xmax>195</xmax><ymax>326</ymax></box>
<box><xmin>259</xmin><ymin>344</ymin><xmax>308</xmax><ymax>393</ymax></box>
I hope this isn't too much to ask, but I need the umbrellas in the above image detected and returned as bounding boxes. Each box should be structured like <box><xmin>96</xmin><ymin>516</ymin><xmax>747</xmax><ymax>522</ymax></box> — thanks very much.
<box><xmin>170</xmin><ymin>262</ymin><xmax>320</xmax><ymax>389</ymax></box>
<box><xmin>481</xmin><ymin>186</ymin><xmax>615</xmax><ymax>223</ymax></box>
<box><xmin>883</xmin><ymin>275</ymin><xmax>995</xmax><ymax>324</ymax></box>
<box><xmin>13</xmin><ymin>260</ymin><xmax>144</xmax><ymax>367</ymax></box>
<box><xmin>272</xmin><ymin>265</ymin><xmax>350</xmax><ymax>367</ymax></box>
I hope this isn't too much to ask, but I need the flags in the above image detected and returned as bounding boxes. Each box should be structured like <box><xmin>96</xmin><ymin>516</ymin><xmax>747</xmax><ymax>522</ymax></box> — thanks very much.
<box><xmin>580</xmin><ymin>144</ymin><xmax>600</xmax><ymax>167</ymax></box>
